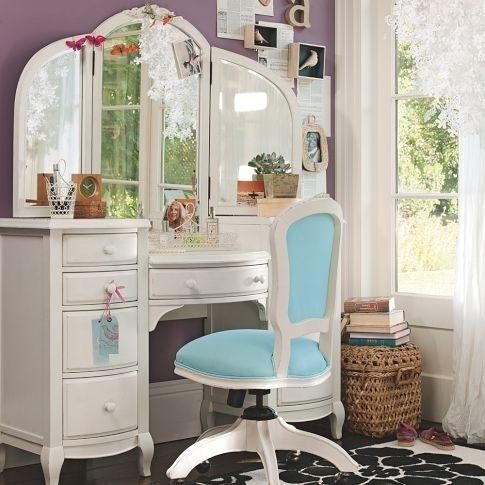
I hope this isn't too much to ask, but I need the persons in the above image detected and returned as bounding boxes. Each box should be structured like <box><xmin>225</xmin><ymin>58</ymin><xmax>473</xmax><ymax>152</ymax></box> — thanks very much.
<box><xmin>168</xmin><ymin>201</ymin><xmax>185</xmax><ymax>232</ymax></box>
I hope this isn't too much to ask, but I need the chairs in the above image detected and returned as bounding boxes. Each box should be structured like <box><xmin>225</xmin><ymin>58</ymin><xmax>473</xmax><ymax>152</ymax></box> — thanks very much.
<box><xmin>166</xmin><ymin>190</ymin><xmax>361</xmax><ymax>485</ymax></box>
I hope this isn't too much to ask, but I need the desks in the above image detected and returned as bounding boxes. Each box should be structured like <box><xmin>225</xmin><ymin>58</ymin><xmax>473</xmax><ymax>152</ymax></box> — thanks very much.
<box><xmin>0</xmin><ymin>219</ymin><xmax>347</xmax><ymax>485</ymax></box>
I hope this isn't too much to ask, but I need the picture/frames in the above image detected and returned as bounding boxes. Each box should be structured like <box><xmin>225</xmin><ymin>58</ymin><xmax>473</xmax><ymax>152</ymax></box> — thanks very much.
<box><xmin>299</xmin><ymin>123</ymin><xmax>329</xmax><ymax>172</ymax></box>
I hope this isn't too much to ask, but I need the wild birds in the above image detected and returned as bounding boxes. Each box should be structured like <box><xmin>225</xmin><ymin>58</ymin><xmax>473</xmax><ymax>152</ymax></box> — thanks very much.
<box><xmin>254</xmin><ymin>28</ymin><xmax>269</xmax><ymax>44</ymax></box>
<box><xmin>299</xmin><ymin>49</ymin><xmax>319</xmax><ymax>72</ymax></box>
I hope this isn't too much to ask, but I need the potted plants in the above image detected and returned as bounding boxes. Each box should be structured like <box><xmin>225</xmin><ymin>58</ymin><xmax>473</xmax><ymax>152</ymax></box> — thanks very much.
<box><xmin>248</xmin><ymin>152</ymin><xmax>263</xmax><ymax>182</ymax></box>
<box><xmin>263</xmin><ymin>152</ymin><xmax>299</xmax><ymax>197</ymax></box>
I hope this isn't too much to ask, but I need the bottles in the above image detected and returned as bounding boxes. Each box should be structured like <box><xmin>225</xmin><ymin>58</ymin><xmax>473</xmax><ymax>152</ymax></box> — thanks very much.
<box><xmin>206</xmin><ymin>207</ymin><xmax>219</xmax><ymax>245</ymax></box>
<box><xmin>159</xmin><ymin>221</ymin><xmax>171</xmax><ymax>246</ymax></box>
<box><xmin>48</xmin><ymin>163</ymin><xmax>75</xmax><ymax>213</ymax></box>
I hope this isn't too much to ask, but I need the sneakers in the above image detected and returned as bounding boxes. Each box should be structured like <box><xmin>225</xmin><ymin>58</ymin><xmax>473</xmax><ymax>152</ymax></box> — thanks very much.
<box><xmin>396</xmin><ymin>422</ymin><xmax>418</xmax><ymax>447</ymax></box>
<box><xmin>419</xmin><ymin>426</ymin><xmax>455</xmax><ymax>451</ymax></box>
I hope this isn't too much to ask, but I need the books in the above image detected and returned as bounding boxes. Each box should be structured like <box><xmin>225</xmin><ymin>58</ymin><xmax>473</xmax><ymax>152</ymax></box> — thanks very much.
<box><xmin>341</xmin><ymin>296</ymin><xmax>410</xmax><ymax>346</ymax></box>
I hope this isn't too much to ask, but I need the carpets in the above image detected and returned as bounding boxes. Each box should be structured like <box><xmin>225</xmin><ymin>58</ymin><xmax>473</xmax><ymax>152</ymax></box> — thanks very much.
<box><xmin>193</xmin><ymin>433</ymin><xmax>485</xmax><ymax>485</ymax></box>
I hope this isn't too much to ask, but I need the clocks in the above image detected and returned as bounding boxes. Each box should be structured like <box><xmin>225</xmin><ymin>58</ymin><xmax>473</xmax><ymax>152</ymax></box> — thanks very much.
<box><xmin>37</xmin><ymin>172</ymin><xmax>56</xmax><ymax>205</ymax></box>
<box><xmin>71</xmin><ymin>172</ymin><xmax>103</xmax><ymax>204</ymax></box>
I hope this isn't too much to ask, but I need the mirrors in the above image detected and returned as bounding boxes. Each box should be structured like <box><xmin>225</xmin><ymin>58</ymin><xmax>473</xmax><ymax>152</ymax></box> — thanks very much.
<box><xmin>12</xmin><ymin>5</ymin><xmax>303</xmax><ymax>228</ymax></box>
